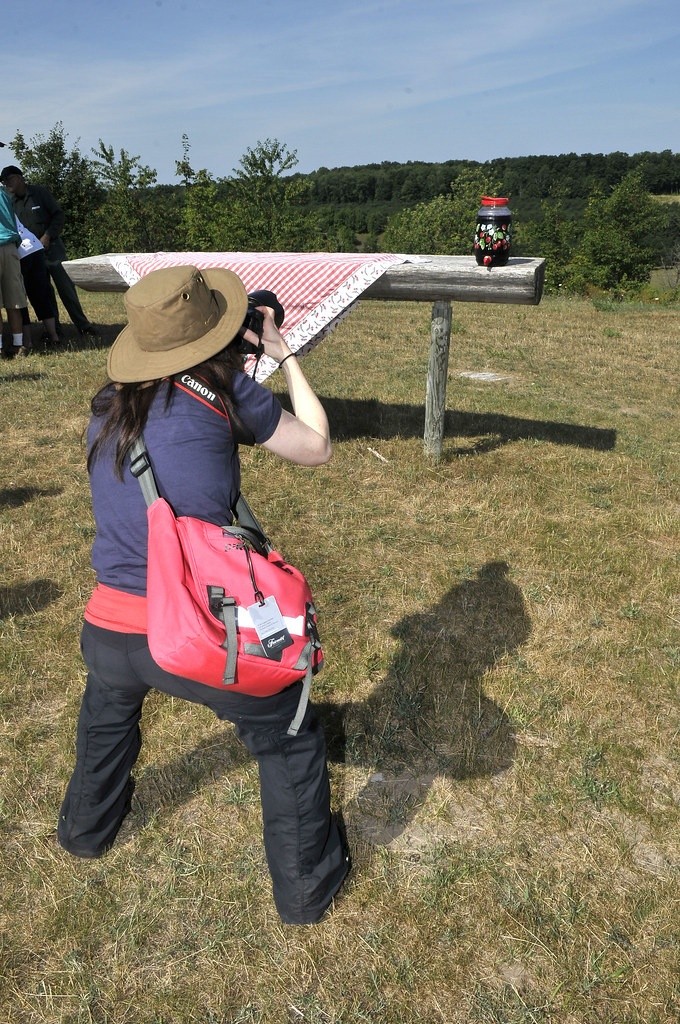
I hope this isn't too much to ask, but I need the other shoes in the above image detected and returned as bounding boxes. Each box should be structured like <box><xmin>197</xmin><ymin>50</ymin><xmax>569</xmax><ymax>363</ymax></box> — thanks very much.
<box><xmin>14</xmin><ymin>345</ymin><xmax>28</xmax><ymax>357</ymax></box>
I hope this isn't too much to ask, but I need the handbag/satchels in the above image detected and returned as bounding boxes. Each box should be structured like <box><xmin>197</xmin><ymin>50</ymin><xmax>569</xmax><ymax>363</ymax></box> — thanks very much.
<box><xmin>13</xmin><ymin>212</ymin><xmax>44</xmax><ymax>260</ymax></box>
<box><xmin>147</xmin><ymin>497</ymin><xmax>324</xmax><ymax>697</ymax></box>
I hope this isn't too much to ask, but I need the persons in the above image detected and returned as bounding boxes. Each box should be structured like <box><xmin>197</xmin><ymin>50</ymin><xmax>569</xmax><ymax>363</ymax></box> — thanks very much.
<box><xmin>0</xmin><ymin>165</ymin><xmax>91</xmax><ymax>353</ymax></box>
<box><xmin>56</xmin><ymin>264</ymin><xmax>351</xmax><ymax>924</ymax></box>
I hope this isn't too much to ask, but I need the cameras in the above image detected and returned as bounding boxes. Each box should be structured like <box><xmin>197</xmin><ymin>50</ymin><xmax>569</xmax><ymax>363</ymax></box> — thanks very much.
<box><xmin>226</xmin><ymin>290</ymin><xmax>284</xmax><ymax>354</ymax></box>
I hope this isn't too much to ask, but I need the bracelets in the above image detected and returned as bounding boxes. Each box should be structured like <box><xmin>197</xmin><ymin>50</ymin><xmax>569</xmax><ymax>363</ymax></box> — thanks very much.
<box><xmin>279</xmin><ymin>353</ymin><xmax>297</xmax><ymax>368</ymax></box>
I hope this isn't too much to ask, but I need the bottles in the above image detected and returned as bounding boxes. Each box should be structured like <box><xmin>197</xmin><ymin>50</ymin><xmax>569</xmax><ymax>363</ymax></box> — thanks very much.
<box><xmin>473</xmin><ymin>195</ymin><xmax>510</xmax><ymax>266</ymax></box>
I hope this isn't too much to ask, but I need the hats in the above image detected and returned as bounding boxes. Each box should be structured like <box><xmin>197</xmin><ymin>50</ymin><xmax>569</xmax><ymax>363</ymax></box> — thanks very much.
<box><xmin>107</xmin><ymin>265</ymin><xmax>248</xmax><ymax>383</ymax></box>
<box><xmin>0</xmin><ymin>165</ymin><xmax>23</xmax><ymax>182</ymax></box>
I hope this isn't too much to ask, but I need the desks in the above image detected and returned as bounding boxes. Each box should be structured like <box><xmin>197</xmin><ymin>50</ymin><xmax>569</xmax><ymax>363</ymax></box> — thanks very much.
<box><xmin>61</xmin><ymin>252</ymin><xmax>547</xmax><ymax>459</ymax></box>
